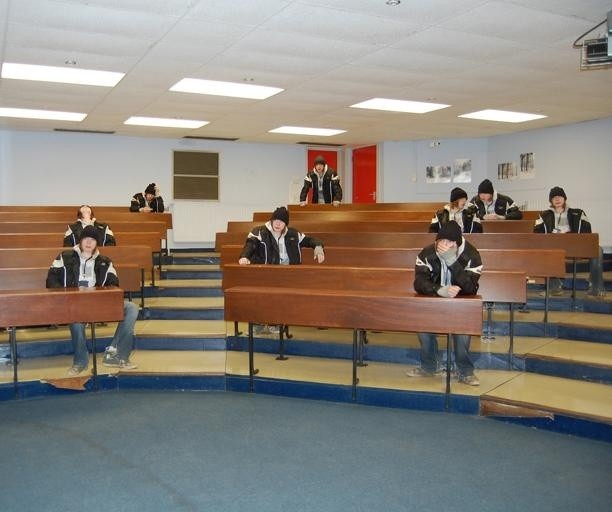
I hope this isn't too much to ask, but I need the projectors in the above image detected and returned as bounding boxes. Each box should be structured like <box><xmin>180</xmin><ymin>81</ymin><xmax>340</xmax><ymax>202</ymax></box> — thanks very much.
<box><xmin>579</xmin><ymin>37</ymin><xmax>612</xmax><ymax>72</ymax></box>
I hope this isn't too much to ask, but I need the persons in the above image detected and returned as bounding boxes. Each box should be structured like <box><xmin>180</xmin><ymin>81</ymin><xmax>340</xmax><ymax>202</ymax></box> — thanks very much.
<box><xmin>45</xmin><ymin>225</ymin><xmax>140</xmax><ymax>376</ymax></box>
<box><xmin>428</xmin><ymin>187</ymin><xmax>483</xmax><ymax>233</ymax></box>
<box><xmin>533</xmin><ymin>187</ymin><xmax>607</xmax><ymax>297</ymax></box>
<box><xmin>63</xmin><ymin>205</ymin><xmax>116</xmax><ymax>247</ymax></box>
<box><xmin>239</xmin><ymin>207</ymin><xmax>326</xmax><ymax>336</ymax></box>
<box><xmin>129</xmin><ymin>183</ymin><xmax>164</xmax><ymax>214</ymax></box>
<box><xmin>299</xmin><ymin>155</ymin><xmax>342</xmax><ymax>208</ymax></box>
<box><xmin>406</xmin><ymin>220</ymin><xmax>483</xmax><ymax>386</ymax></box>
<box><xmin>470</xmin><ymin>179</ymin><xmax>522</xmax><ymax>221</ymax></box>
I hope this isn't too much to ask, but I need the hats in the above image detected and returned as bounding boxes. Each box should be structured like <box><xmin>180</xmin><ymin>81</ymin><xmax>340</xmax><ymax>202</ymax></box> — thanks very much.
<box><xmin>549</xmin><ymin>187</ymin><xmax>567</xmax><ymax>202</ymax></box>
<box><xmin>436</xmin><ymin>220</ymin><xmax>462</xmax><ymax>247</ymax></box>
<box><xmin>271</xmin><ymin>207</ymin><xmax>289</xmax><ymax>226</ymax></box>
<box><xmin>313</xmin><ymin>155</ymin><xmax>324</xmax><ymax>167</ymax></box>
<box><xmin>80</xmin><ymin>225</ymin><xmax>99</xmax><ymax>243</ymax></box>
<box><xmin>145</xmin><ymin>183</ymin><xmax>155</xmax><ymax>195</ymax></box>
<box><xmin>478</xmin><ymin>179</ymin><xmax>494</xmax><ymax>195</ymax></box>
<box><xmin>450</xmin><ymin>187</ymin><xmax>468</xmax><ymax>202</ymax></box>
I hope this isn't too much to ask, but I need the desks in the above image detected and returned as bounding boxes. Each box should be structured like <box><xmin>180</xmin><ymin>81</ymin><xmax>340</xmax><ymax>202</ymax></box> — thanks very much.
<box><xmin>1</xmin><ymin>202</ymin><xmax>174</xmax><ymax>392</ymax></box>
<box><xmin>215</xmin><ymin>201</ymin><xmax>601</xmax><ymax>414</ymax></box>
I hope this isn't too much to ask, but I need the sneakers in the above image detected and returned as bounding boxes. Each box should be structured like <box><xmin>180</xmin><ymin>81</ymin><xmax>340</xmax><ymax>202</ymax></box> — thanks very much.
<box><xmin>245</xmin><ymin>325</ymin><xmax>279</xmax><ymax>336</ymax></box>
<box><xmin>67</xmin><ymin>365</ymin><xmax>87</xmax><ymax>377</ymax></box>
<box><xmin>459</xmin><ymin>375</ymin><xmax>480</xmax><ymax>387</ymax></box>
<box><xmin>405</xmin><ymin>368</ymin><xmax>438</xmax><ymax>378</ymax></box>
<box><xmin>539</xmin><ymin>286</ymin><xmax>565</xmax><ymax>296</ymax></box>
<box><xmin>102</xmin><ymin>346</ymin><xmax>137</xmax><ymax>369</ymax></box>
<box><xmin>586</xmin><ymin>285</ymin><xmax>612</xmax><ymax>300</ymax></box>
